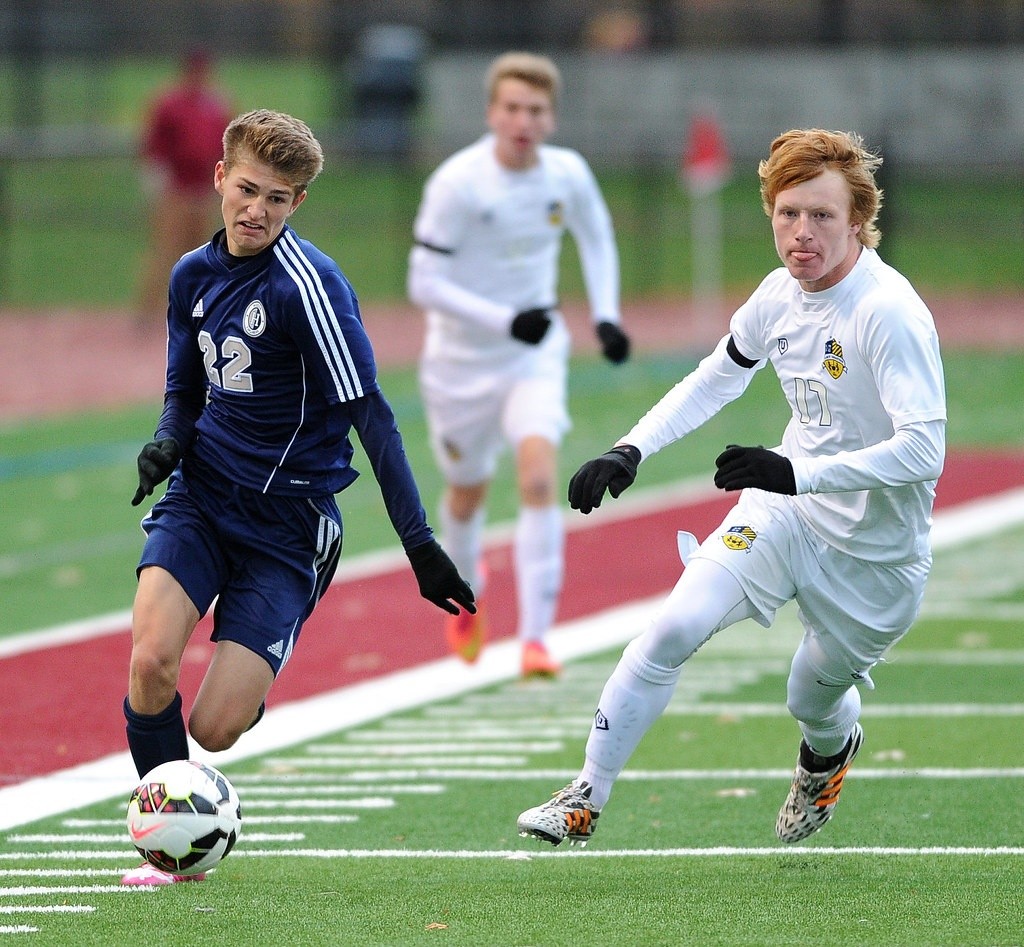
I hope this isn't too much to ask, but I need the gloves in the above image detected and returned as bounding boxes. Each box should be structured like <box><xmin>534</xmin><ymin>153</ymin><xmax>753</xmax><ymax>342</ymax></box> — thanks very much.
<box><xmin>129</xmin><ymin>438</ymin><xmax>182</xmax><ymax>508</ymax></box>
<box><xmin>596</xmin><ymin>322</ymin><xmax>631</xmax><ymax>366</ymax></box>
<box><xmin>404</xmin><ymin>541</ymin><xmax>478</xmax><ymax>620</ymax></box>
<box><xmin>712</xmin><ymin>443</ymin><xmax>798</xmax><ymax>498</ymax></box>
<box><xmin>511</xmin><ymin>308</ymin><xmax>553</xmax><ymax>345</ymax></box>
<box><xmin>567</xmin><ymin>445</ymin><xmax>643</xmax><ymax>516</ymax></box>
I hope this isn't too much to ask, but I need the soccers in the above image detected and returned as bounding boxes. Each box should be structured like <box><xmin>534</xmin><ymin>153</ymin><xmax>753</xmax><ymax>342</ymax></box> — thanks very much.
<box><xmin>124</xmin><ymin>760</ymin><xmax>243</xmax><ymax>877</ymax></box>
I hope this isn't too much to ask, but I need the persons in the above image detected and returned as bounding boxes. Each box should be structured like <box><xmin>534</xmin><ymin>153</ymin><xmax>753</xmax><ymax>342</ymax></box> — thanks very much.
<box><xmin>329</xmin><ymin>0</ymin><xmax>642</xmax><ymax>179</ymax></box>
<box><xmin>118</xmin><ymin>110</ymin><xmax>479</xmax><ymax>887</ymax></box>
<box><xmin>518</xmin><ymin>131</ymin><xmax>946</xmax><ymax>843</ymax></box>
<box><xmin>408</xmin><ymin>54</ymin><xmax>630</xmax><ymax>677</ymax></box>
<box><xmin>132</xmin><ymin>43</ymin><xmax>250</xmax><ymax>352</ymax></box>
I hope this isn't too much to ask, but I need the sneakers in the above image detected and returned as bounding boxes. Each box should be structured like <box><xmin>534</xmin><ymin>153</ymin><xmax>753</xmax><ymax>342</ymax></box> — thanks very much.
<box><xmin>517</xmin><ymin>637</ymin><xmax>560</xmax><ymax>678</ymax></box>
<box><xmin>774</xmin><ymin>721</ymin><xmax>866</xmax><ymax>845</ymax></box>
<box><xmin>515</xmin><ymin>785</ymin><xmax>602</xmax><ymax>846</ymax></box>
<box><xmin>120</xmin><ymin>859</ymin><xmax>209</xmax><ymax>888</ymax></box>
<box><xmin>443</xmin><ymin>601</ymin><xmax>483</xmax><ymax>664</ymax></box>
